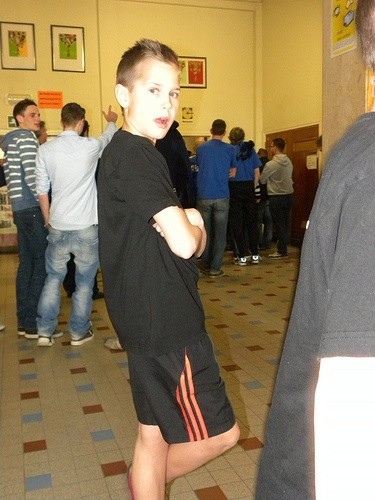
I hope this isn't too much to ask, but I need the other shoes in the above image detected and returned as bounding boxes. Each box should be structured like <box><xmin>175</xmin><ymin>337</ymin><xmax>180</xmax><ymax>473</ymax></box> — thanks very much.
<box><xmin>251</xmin><ymin>255</ymin><xmax>260</xmax><ymax>264</ymax></box>
<box><xmin>233</xmin><ymin>257</ymin><xmax>247</xmax><ymax>266</ymax></box>
<box><xmin>268</xmin><ymin>251</ymin><xmax>288</xmax><ymax>258</ymax></box>
<box><xmin>204</xmin><ymin>267</ymin><xmax>225</xmax><ymax>279</ymax></box>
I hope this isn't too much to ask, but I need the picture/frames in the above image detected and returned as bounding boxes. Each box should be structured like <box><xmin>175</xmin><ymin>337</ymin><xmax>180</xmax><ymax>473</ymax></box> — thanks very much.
<box><xmin>0</xmin><ymin>22</ymin><xmax>36</xmax><ymax>70</ymax></box>
<box><xmin>51</xmin><ymin>25</ymin><xmax>85</xmax><ymax>73</ymax></box>
<box><xmin>178</xmin><ymin>56</ymin><xmax>207</xmax><ymax>89</ymax></box>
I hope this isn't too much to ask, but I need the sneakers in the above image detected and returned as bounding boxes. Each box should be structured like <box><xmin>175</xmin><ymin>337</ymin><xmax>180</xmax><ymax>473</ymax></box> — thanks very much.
<box><xmin>37</xmin><ymin>333</ymin><xmax>55</xmax><ymax>347</ymax></box>
<box><xmin>71</xmin><ymin>329</ymin><xmax>94</xmax><ymax>347</ymax></box>
<box><xmin>25</xmin><ymin>328</ymin><xmax>63</xmax><ymax>339</ymax></box>
<box><xmin>17</xmin><ymin>327</ymin><xmax>26</xmax><ymax>336</ymax></box>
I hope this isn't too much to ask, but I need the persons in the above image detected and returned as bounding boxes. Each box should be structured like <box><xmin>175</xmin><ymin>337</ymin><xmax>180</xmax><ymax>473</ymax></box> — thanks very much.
<box><xmin>35</xmin><ymin>102</ymin><xmax>117</xmax><ymax>345</ymax></box>
<box><xmin>63</xmin><ymin>120</ymin><xmax>104</xmax><ymax>300</ymax></box>
<box><xmin>155</xmin><ymin>120</ymin><xmax>294</xmax><ymax>278</ymax></box>
<box><xmin>34</xmin><ymin>120</ymin><xmax>47</xmax><ymax>144</ymax></box>
<box><xmin>0</xmin><ymin>163</ymin><xmax>7</xmax><ymax>330</ymax></box>
<box><xmin>315</xmin><ymin>135</ymin><xmax>322</xmax><ymax>151</ymax></box>
<box><xmin>0</xmin><ymin>99</ymin><xmax>63</xmax><ymax>338</ymax></box>
<box><xmin>254</xmin><ymin>0</ymin><xmax>375</xmax><ymax>500</ymax></box>
<box><xmin>95</xmin><ymin>40</ymin><xmax>242</xmax><ymax>500</ymax></box>
